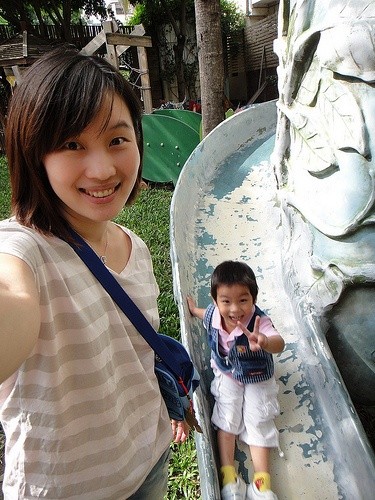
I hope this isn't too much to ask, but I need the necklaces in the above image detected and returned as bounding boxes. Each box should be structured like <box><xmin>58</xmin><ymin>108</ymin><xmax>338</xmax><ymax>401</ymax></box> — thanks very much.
<box><xmin>78</xmin><ymin>230</ymin><xmax>108</xmax><ymax>264</ymax></box>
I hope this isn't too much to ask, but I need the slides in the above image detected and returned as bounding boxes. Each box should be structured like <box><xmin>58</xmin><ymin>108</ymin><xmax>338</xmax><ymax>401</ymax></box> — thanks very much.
<box><xmin>166</xmin><ymin>94</ymin><xmax>375</xmax><ymax>500</ymax></box>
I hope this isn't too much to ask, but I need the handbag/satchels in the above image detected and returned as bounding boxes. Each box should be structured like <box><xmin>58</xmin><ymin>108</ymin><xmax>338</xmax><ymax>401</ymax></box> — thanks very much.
<box><xmin>154</xmin><ymin>332</ymin><xmax>203</xmax><ymax>434</ymax></box>
<box><xmin>231</xmin><ymin>348</ymin><xmax>275</xmax><ymax>383</ymax></box>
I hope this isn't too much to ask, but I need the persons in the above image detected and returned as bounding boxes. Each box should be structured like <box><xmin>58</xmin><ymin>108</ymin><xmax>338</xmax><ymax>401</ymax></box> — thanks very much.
<box><xmin>0</xmin><ymin>50</ymin><xmax>191</xmax><ymax>500</ymax></box>
<box><xmin>186</xmin><ymin>261</ymin><xmax>285</xmax><ymax>500</ymax></box>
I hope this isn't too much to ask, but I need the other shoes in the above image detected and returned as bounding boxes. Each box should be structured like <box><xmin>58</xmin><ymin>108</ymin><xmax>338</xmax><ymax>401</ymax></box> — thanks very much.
<box><xmin>246</xmin><ymin>481</ymin><xmax>278</xmax><ymax>500</ymax></box>
<box><xmin>221</xmin><ymin>476</ymin><xmax>246</xmax><ymax>500</ymax></box>
